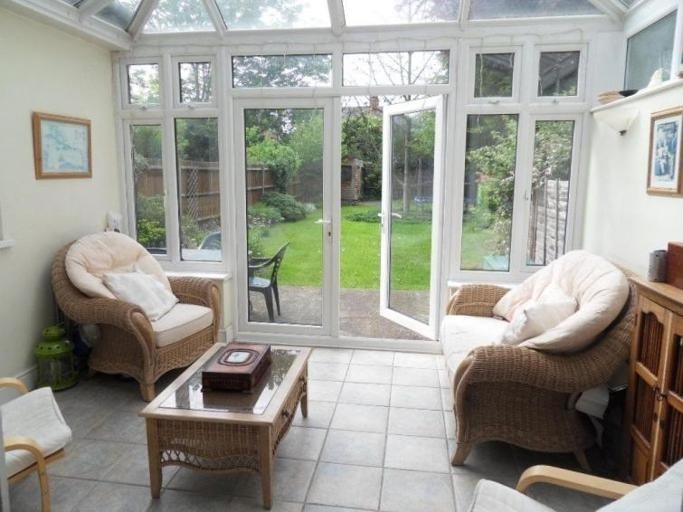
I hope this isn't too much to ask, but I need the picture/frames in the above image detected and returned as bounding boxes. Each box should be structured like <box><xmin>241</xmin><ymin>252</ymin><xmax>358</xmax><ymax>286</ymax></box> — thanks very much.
<box><xmin>646</xmin><ymin>105</ymin><xmax>683</xmax><ymax>197</ymax></box>
<box><xmin>33</xmin><ymin>111</ymin><xmax>92</xmax><ymax>179</ymax></box>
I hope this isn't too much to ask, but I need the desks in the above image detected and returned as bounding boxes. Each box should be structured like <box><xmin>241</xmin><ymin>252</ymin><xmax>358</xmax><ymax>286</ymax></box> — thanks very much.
<box><xmin>151</xmin><ymin>249</ymin><xmax>253</xmax><ymax>314</ymax></box>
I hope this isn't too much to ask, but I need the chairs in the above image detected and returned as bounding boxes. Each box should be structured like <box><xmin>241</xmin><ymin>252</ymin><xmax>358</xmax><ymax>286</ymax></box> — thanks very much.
<box><xmin>146</xmin><ymin>248</ymin><xmax>183</xmax><ymax>261</ymax></box>
<box><xmin>51</xmin><ymin>231</ymin><xmax>222</xmax><ymax>402</ymax></box>
<box><xmin>198</xmin><ymin>231</ymin><xmax>221</xmax><ymax>250</ymax></box>
<box><xmin>0</xmin><ymin>376</ymin><xmax>73</xmax><ymax>512</ymax></box>
<box><xmin>469</xmin><ymin>457</ymin><xmax>683</xmax><ymax>512</ymax></box>
<box><xmin>248</xmin><ymin>242</ymin><xmax>289</xmax><ymax>322</ymax></box>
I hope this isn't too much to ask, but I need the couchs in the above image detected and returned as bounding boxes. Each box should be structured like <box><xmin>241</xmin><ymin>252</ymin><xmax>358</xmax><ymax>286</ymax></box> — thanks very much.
<box><xmin>439</xmin><ymin>249</ymin><xmax>641</xmax><ymax>474</ymax></box>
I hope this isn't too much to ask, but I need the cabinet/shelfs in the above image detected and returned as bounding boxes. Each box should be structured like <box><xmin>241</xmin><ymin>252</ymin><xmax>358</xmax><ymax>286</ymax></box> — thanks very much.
<box><xmin>619</xmin><ymin>276</ymin><xmax>683</xmax><ymax>486</ymax></box>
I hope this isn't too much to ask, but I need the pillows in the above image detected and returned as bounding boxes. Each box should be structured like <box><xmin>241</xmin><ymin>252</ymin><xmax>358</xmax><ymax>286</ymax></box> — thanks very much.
<box><xmin>102</xmin><ymin>271</ymin><xmax>180</xmax><ymax>321</ymax></box>
<box><xmin>500</xmin><ymin>281</ymin><xmax>578</xmax><ymax>344</ymax></box>
